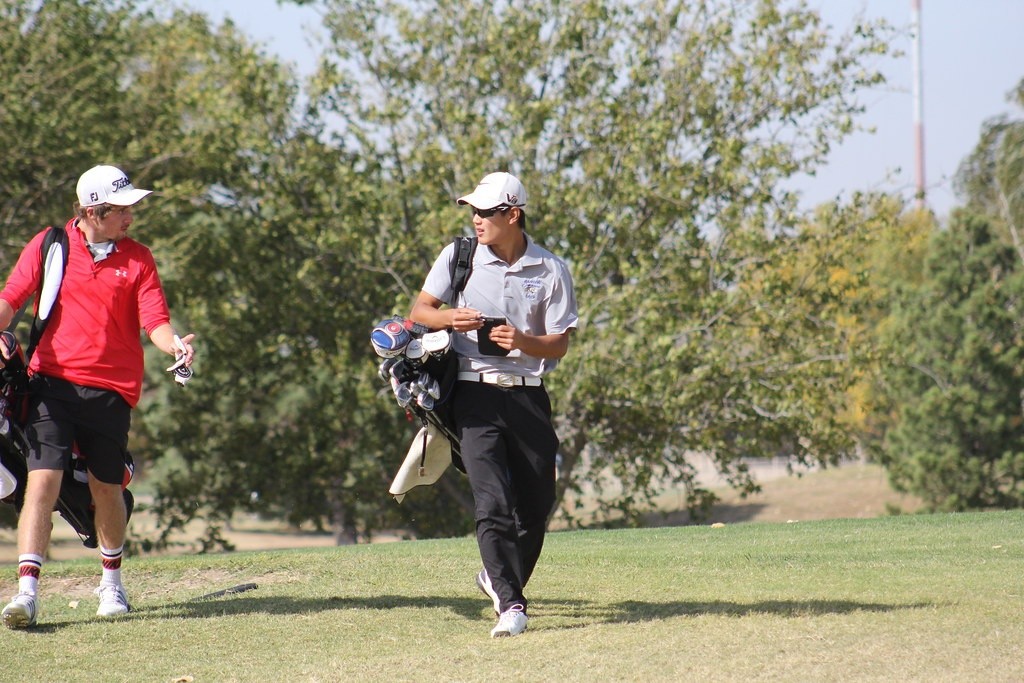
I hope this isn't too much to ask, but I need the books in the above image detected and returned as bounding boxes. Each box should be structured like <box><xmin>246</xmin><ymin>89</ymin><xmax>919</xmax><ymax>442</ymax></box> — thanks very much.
<box><xmin>474</xmin><ymin>316</ymin><xmax>510</xmax><ymax>357</ymax></box>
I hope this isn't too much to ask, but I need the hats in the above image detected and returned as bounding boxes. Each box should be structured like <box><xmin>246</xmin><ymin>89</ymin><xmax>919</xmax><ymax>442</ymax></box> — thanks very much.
<box><xmin>456</xmin><ymin>172</ymin><xmax>526</xmax><ymax>210</ymax></box>
<box><xmin>76</xmin><ymin>164</ymin><xmax>155</xmax><ymax>207</ymax></box>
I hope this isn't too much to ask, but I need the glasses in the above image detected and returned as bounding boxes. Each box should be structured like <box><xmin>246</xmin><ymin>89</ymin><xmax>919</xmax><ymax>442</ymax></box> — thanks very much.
<box><xmin>472</xmin><ymin>205</ymin><xmax>512</xmax><ymax>218</ymax></box>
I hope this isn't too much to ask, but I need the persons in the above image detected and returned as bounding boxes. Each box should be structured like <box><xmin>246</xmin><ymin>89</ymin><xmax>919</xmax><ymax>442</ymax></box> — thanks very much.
<box><xmin>409</xmin><ymin>171</ymin><xmax>580</xmax><ymax>637</ymax></box>
<box><xmin>0</xmin><ymin>165</ymin><xmax>195</xmax><ymax>629</ymax></box>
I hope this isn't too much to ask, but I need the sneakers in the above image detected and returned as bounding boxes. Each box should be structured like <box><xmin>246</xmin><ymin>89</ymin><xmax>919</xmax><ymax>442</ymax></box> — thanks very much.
<box><xmin>476</xmin><ymin>568</ymin><xmax>501</xmax><ymax>617</ymax></box>
<box><xmin>1</xmin><ymin>592</ymin><xmax>40</xmax><ymax>629</ymax></box>
<box><xmin>490</xmin><ymin>604</ymin><xmax>529</xmax><ymax>638</ymax></box>
<box><xmin>93</xmin><ymin>584</ymin><xmax>130</xmax><ymax>619</ymax></box>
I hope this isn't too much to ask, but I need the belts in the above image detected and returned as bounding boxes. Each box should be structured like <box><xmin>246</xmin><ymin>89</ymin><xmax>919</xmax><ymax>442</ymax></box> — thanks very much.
<box><xmin>456</xmin><ymin>371</ymin><xmax>542</xmax><ymax>388</ymax></box>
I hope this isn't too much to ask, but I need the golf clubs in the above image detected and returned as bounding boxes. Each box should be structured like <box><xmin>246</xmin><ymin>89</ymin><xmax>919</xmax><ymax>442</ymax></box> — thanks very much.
<box><xmin>369</xmin><ymin>314</ymin><xmax>451</xmax><ymax>411</ymax></box>
<box><xmin>0</xmin><ymin>413</ymin><xmax>12</xmax><ymax>439</ymax></box>
